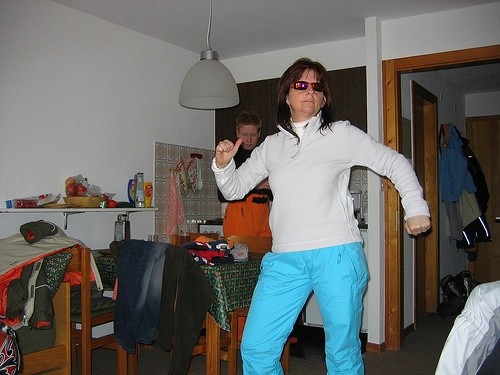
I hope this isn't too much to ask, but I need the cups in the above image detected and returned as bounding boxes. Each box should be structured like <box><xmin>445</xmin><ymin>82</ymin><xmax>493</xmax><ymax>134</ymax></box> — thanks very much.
<box><xmin>178</xmin><ymin>226</ymin><xmax>191</xmax><ymax>246</ymax></box>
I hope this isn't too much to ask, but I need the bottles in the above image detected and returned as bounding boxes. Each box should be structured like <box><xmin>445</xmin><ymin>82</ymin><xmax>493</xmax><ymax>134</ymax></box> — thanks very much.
<box><xmin>135</xmin><ymin>172</ymin><xmax>146</xmax><ymax>207</ymax></box>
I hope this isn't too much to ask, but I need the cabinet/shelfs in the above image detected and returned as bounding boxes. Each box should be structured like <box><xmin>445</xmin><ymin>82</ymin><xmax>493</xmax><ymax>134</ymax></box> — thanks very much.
<box><xmin>0</xmin><ymin>208</ymin><xmax>158</xmax><ymax>231</ymax></box>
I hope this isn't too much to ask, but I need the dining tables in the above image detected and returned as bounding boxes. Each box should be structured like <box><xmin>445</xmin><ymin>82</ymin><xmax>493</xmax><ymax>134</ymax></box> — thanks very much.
<box><xmin>91</xmin><ymin>247</ymin><xmax>272</xmax><ymax>375</ymax></box>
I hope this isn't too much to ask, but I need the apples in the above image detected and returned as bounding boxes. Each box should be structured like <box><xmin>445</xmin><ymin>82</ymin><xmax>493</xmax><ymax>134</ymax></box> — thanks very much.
<box><xmin>64</xmin><ymin>177</ymin><xmax>86</xmax><ymax>196</ymax></box>
<box><xmin>108</xmin><ymin>200</ymin><xmax>116</xmax><ymax>208</ymax></box>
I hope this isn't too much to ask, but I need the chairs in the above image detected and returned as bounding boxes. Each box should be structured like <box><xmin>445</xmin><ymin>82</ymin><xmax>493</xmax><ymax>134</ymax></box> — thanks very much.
<box><xmin>166</xmin><ymin>233</ymin><xmax>293</xmax><ymax>375</ymax></box>
<box><xmin>0</xmin><ymin>246</ymin><xmax>138</xmax><ymax>375</ymax></box>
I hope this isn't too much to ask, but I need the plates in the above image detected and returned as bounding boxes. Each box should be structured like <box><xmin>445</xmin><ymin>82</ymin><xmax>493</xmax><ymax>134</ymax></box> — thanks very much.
<box><xmin>61</xmin><ymin>196</ymin><xmax>107</xmax><ymax>207</ymax></box>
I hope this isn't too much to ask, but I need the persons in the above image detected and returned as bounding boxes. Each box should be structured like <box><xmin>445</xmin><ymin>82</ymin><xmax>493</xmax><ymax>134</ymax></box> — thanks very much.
<box><xmin>211</xmin><ymin>58</ymin><xmax>430</xmax><ymax>375</ymax></box>
<box><xmin>217</xmin><ymin>111</ymin><xmax>273</xmax><ymax>237</ymax></box>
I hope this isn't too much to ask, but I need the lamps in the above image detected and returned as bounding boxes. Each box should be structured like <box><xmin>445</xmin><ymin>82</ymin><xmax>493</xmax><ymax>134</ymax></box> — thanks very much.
<box><xmin>180</xmin><ymin>0</ymin><xmax>239</xmax><ymax>109</ymax></box>
